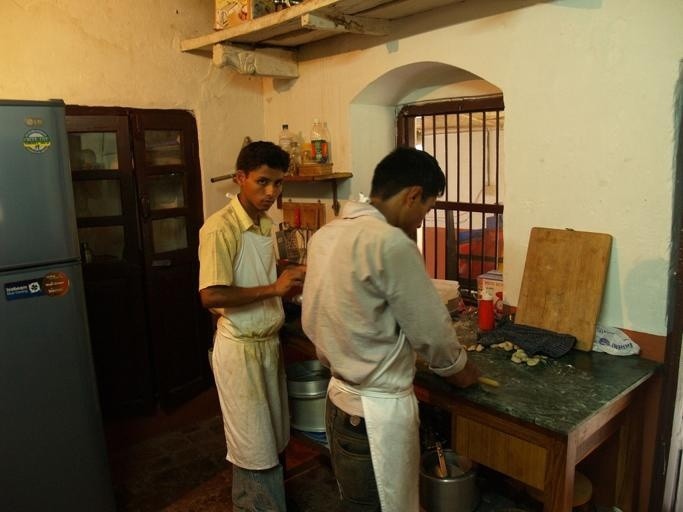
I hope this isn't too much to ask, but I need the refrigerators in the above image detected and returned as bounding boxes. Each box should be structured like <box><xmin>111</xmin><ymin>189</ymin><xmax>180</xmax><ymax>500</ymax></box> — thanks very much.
<box><xmin>1</xmin><ymin>103</ymin><xmax>114</xmax><ymax>510</ymax></box>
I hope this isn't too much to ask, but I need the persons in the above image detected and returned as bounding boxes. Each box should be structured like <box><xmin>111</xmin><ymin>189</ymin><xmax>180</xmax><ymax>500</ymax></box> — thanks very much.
<box><xmin>299</xmin><ymin>142</ymin><xmax>478</xmax><ymax>512</ymax></box>
<box><xmin>198</xmin><ymin>141</ymin><xmax>309</xmax><ymax>511</ymax></box>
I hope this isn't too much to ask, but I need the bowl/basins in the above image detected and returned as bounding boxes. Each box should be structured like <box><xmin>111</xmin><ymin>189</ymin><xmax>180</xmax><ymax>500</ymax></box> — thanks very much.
<box><xmin>287</xmin><ymin>359</ymin><xmax>332</xmax><ymax>400</ymax></box>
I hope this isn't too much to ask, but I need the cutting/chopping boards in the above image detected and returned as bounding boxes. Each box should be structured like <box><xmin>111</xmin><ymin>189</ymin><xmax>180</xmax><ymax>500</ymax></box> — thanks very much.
<box><xmin>512</xmin><ymin>226</ymin><xmax>612</xmax><ymax>354</ymax></box>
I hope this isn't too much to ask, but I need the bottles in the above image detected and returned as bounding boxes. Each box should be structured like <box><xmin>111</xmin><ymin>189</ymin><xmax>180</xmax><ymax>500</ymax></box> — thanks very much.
<box><xmin>309</xmin><ymin>117</ymin><xmax>329</xmax><ymax>164</ymax></box>
<box><xmin>278</xmin><ymin>123</ymin><xmax>301</xmax><ymax>179</ymax></box>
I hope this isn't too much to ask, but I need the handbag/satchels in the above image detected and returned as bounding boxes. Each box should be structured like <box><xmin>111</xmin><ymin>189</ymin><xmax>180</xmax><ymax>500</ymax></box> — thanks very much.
<box><xmin>276</xmin><ymin>222</ymin><xmax>305</xmax><ymax>264</ymax></box>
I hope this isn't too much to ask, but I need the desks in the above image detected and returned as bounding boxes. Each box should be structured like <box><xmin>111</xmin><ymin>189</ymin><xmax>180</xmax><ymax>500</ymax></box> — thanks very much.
<box><xmin>405</xmin><ymin>316</ymin><xmax>663</xmax><ymax>511</ymax></box>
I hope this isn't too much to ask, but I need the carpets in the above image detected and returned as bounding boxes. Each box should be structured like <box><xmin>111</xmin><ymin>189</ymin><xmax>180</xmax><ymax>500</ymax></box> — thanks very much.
<box><xmin>281</xmin><ymin>453</ymin><xmax>544</xmax><ymax>510</ymax></box>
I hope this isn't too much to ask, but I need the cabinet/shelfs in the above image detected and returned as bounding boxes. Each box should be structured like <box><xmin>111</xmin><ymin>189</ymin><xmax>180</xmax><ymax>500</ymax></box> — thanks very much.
<box><xmin>65</xmin><ymin>106</ymin><xmax>215</xmax><ymax>420</ymax></box>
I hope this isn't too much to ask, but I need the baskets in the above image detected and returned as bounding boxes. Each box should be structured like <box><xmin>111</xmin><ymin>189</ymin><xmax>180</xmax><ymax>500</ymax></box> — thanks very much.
<box><xmin>298</xmin><ymin>164</ymin><xmax>332</xmax><ymax>177</ymax></box>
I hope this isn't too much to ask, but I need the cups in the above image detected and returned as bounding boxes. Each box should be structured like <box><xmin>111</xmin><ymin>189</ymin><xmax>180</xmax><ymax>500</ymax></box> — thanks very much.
<box><xmin>477</xmin><ymin>297</ymin><xmax>494</xmax><ymax>332</ymax></box>
